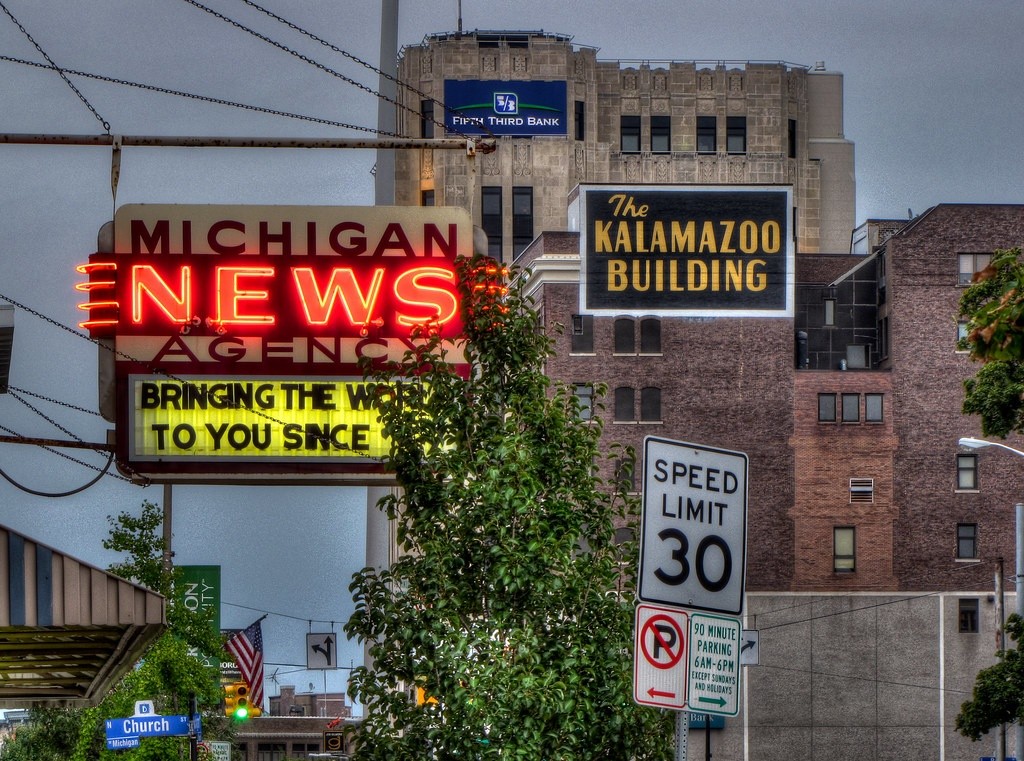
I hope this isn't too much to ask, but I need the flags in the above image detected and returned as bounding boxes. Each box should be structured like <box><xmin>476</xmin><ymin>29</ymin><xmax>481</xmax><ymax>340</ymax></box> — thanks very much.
<box><xmin>224</xmin><ymin>620</ymin><xmax>264</xmax><ymax>714</ymax></box>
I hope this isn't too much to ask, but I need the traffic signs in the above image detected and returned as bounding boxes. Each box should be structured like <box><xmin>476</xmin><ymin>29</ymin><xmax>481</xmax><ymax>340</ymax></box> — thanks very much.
<box><xmin>685</xmin><ymin>612</ymin><xmax>742</xmax><ymax>720</ymax></box>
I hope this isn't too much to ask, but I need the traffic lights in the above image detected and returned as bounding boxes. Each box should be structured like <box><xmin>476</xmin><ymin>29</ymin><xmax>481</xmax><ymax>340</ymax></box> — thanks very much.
<box><xmin>224</xmin><ymin>685</ymin><xmax>236</xmax><ymax>718</ymax></box>
<box><xmin>249</xmin><ymin>705</ymin><xmax>262</xmax><ymax>719</ymax></box>
<box><xmin>233</xmin><ymin>682</ymin><xmax>252</xmax><ymax>721</ymax></box>
